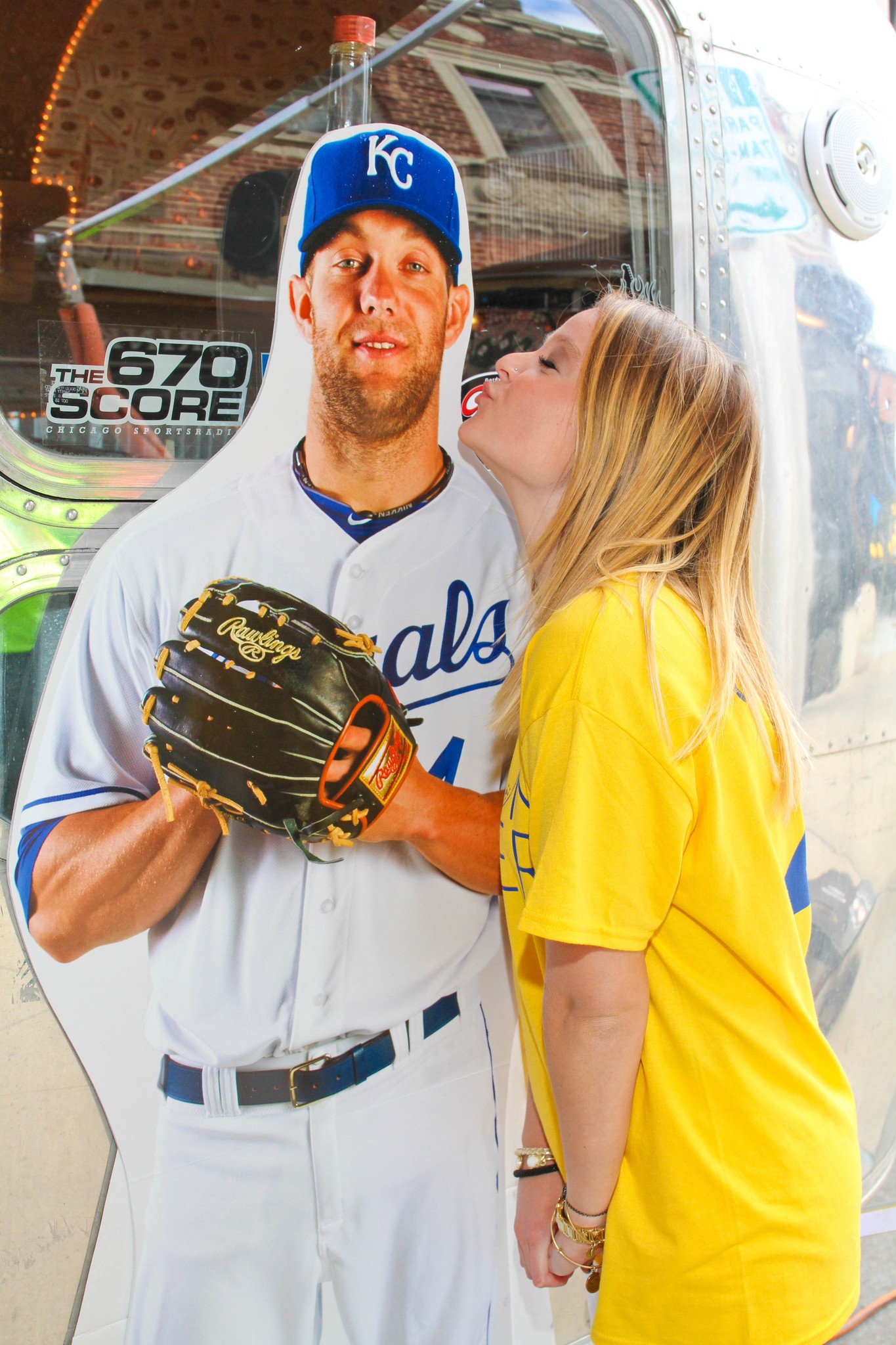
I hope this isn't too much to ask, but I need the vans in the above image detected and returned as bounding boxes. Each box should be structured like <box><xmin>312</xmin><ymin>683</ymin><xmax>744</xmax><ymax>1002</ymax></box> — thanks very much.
<box><xmin>0</xmin><ymin>1</ymin><xmax>894</xmax><ymax>1342</ymax></box>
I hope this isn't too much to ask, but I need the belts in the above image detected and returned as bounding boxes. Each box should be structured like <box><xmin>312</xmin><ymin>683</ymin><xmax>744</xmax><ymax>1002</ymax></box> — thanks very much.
<box><xmin>156</xmin><ymin>992</ymin><xmax>460</xmax><ymax>1110</ymax></box>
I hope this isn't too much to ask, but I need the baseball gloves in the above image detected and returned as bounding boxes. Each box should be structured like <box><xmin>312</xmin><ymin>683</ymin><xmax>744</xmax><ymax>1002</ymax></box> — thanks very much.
<box><xmin>142</xmin><ymin>580</ymin><xmax>418</xmax><ymax>846</ymax></box>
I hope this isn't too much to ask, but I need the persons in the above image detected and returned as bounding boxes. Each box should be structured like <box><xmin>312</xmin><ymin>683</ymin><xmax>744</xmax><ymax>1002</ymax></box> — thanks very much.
<box><xmin>458</xmin><ymin>291</ymin><xmax>862</xmax><ymax>1345</ymax></box>
<box><xmin>17</xmin><ymin>129</ymin><xmax>539</xmax><ymax>1345</ymax></box>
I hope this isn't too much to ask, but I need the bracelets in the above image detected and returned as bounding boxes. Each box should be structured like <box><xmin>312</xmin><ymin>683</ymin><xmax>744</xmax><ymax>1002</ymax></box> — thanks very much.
<box><xmin>510</xmin><ymin>1146</ymin><xmax>559</xmax><ymax>1177</ymax></box>
<box><xmin>553</xmin><ymin>1182</ymin><xmax>608</xmax><ymax>1294</ymax></box>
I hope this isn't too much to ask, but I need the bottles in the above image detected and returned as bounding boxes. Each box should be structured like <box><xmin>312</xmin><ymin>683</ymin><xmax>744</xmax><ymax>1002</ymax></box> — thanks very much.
<box><xmin>325</xmin><ymin>15</ymin><xmax>376</xmax><ymax>132</ymax></box>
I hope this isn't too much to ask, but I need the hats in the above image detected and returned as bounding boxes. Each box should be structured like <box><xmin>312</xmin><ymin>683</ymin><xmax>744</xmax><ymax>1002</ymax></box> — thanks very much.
<box><xmin>299</xmin><ymin>129</ymin><xmax>463</xmax><ymax>287</ymax></box>
